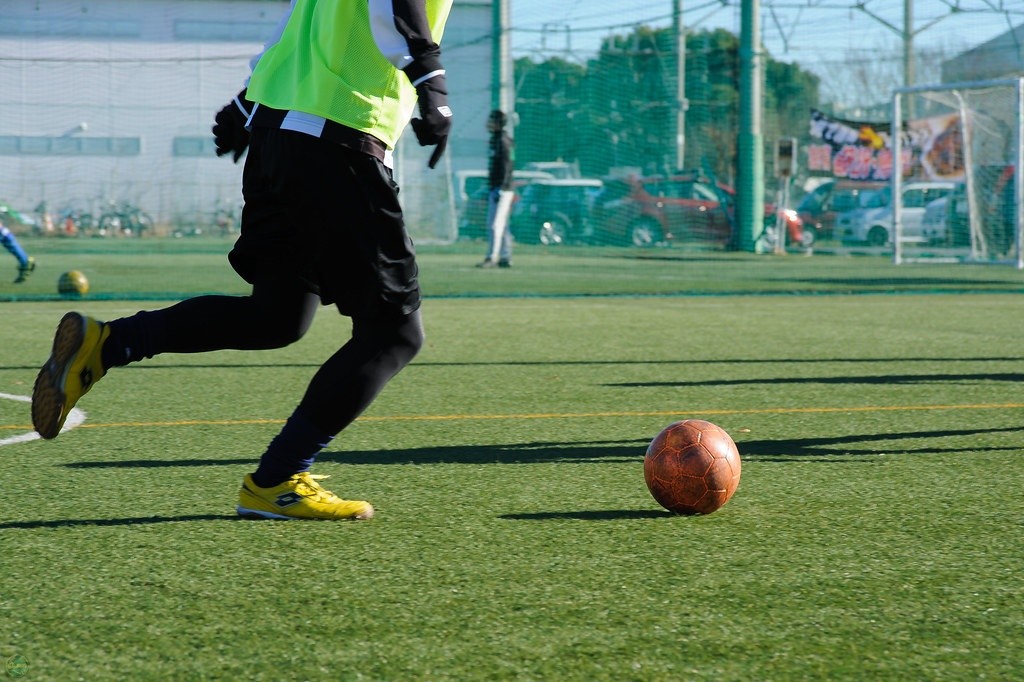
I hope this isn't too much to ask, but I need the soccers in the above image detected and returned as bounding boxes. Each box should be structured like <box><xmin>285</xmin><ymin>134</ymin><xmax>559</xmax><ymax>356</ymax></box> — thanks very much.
<box><xmin>642</xmin><ymin>418</ymin><xmax>742</xmax><ymax>516</ymax></box>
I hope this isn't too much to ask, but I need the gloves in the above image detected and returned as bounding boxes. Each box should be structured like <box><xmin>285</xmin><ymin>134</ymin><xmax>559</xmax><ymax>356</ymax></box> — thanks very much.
<box><xmin>491</xmin><ymin>187</ymin><xmax>503</xmax><ymax>202</ymax></box>
<box><xmin>402</xmin><ymin>54</ymin><xmax>453</xmax><ymax>169</ymax></box>
<box><xmin>213</xmin><ymin>88</ymin><xmax>256</xmax><ymax>162</ymax></box>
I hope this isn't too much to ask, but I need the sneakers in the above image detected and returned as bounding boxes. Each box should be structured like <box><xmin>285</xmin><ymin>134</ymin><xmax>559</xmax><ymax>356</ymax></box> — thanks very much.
<box><xmin>237</xmin><ymin>472</ymin><xmax>373</xmax><ymax>521</ymax></box>
<box><xmin>14</xmin><ymin>256</ymin><xmax>34</xmax><ymax>284</ymax></box>
<box><xmin>32</xmin><ymin>310</ymin><xmax>113</xmax><ymax>441</ymax></box>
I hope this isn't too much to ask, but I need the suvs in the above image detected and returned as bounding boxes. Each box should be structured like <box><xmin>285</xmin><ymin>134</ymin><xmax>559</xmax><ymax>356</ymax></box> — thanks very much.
<box><xmin>851</xmin><ymin>180</ymin><xmax>959</xmax><ymax>246</ymax></box>
<box><xmin>593</xmin><ymin>176</ymin><xmax>805</xmax><ymax>250</ymax></box>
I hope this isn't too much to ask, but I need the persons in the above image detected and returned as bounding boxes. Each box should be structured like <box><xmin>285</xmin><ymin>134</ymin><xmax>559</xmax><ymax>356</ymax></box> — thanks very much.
<box><xmin>0</xmin><ymin>222</ymin><xmax>36</xmax><ymax>283</ymax></box>
<box><xmin>474</xmin><ymin>109</ymin><xmax>518</xmax><ymax>268</ymax></box>
<box><xmin>31</xmin><ymin>0</ymin><xmax>454</xmax><ymax>519</ymax></box>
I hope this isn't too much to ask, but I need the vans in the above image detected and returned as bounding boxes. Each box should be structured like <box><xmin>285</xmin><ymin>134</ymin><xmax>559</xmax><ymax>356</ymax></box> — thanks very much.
<box><xmin>798</xmin><ymin>180</ymin><xmax>888</xmax><ymax>240</ymax></box>
<box><xmin>451</xmin><ymin>171</ymin><xmax>556</xmax><ymax>242</ymax></box>
<box><xmin>511</xmin><ymin>180</ymin><xmax>599</xmax><ymax>247</ymax></box>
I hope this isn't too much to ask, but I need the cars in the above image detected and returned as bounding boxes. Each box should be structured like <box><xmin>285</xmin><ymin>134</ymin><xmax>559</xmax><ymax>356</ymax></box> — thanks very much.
<box><xmin>923</xmin><ymin>196</ymin><xmax>956</xmax><ymax>245</ymax></box>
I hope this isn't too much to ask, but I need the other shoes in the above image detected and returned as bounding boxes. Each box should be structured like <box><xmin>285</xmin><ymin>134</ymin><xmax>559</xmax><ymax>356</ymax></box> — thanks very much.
<box><xmin>496</xmin><ymin>259</ymin><xmax>512</xmax><ymax>268</ymax></box>
<box><xmin>477</xmin><ymin>258</ymin><xmax>496</xmax><ymax>268</ymax></box>
